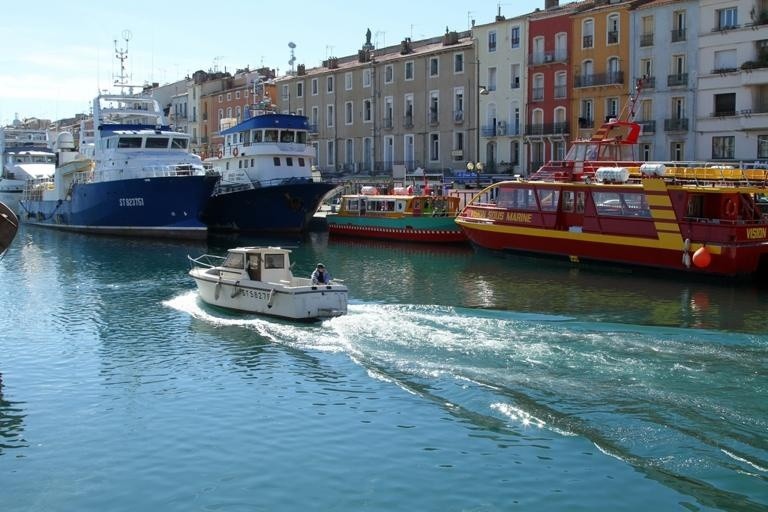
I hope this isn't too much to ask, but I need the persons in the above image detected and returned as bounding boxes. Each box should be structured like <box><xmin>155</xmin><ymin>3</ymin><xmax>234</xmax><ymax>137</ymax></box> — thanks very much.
<box><xmin>316</xmin><ymin>206</ymin><xmax>322</xmax><ymax>212</ymax></box>
<box><xmin>430</xmin><ymin>189</ymin><xmax>435</xmax><ymax>194</ymax></box>
<box><xmin>336</xmin><ymin>194</ymin><xmax>340</xmax><ymax>204</ymax></box>
<box><xmin>310</xmin><ymin>263</ymin><xmax>330</xmax><ymax>285</ymax></box>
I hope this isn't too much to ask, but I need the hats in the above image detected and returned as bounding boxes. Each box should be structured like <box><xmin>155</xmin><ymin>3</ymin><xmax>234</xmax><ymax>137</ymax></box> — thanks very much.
<box><xmin>317</xmin><ymin>265</ymin><xmax>324</xmax><ymax>269</ymax></box>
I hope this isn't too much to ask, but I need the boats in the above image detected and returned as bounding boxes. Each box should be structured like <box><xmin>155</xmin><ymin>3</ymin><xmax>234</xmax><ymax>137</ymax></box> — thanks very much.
<box><xmin>186</xmin><ymin>246</ymin><xmax>347</xmax><ymax>319</ymax></box>
<box><xmin>326</xmin><ymin>193</ymin><xmax>470</xmax><ymax>244</ymax></box>
<box><xmin>454</xmin><ymin>120</ymin><xmax>768</xmax><ymax>274</ymax></box>
